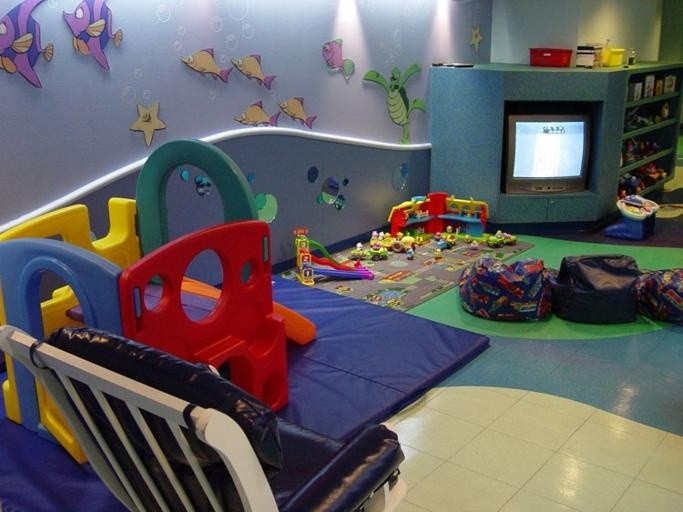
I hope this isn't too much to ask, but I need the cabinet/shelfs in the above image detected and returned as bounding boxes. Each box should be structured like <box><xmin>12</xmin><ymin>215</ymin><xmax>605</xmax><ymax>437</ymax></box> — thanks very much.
<box><xmin>618</xmin><ymin>60</ymin><xmax>683</xmax><ymax>198</ymax></box>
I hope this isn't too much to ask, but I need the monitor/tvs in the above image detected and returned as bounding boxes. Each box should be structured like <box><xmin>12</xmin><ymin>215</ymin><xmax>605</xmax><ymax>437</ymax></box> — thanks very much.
<box><xmin>505</xmin><ymin>114</ymin><xmax>590</xmax><ymax>194</ymax></box>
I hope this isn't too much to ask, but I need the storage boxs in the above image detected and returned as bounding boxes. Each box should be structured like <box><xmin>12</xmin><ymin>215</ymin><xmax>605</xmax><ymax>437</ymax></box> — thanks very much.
<box><xmin>604</xmin><ymin>48</ymin><xmax>625</xmax><ymax>67</ymax></box>
<box><xmin>528</xmin><ymin>47</ymin><xmax>572</xmax><ymax>67</ymax></box>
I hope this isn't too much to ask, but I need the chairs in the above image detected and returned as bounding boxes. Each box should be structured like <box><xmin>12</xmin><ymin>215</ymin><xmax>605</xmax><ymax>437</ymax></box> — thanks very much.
<box><xmin>0</xmin><ymin>322</ymin><xmax>406</xmax><ymax>512</ymax></box>
